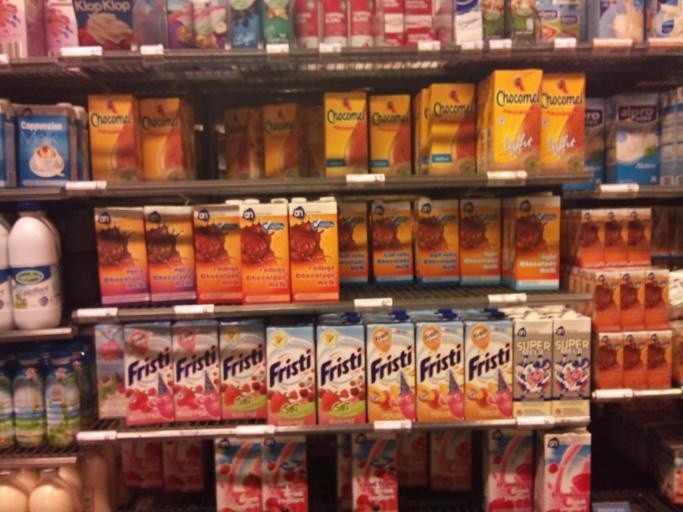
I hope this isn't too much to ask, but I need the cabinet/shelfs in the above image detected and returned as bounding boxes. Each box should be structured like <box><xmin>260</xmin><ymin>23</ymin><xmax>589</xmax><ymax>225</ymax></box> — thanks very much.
<box><xmin>0</xmin><ymin>0</ymin><xmax>683</xmax><ymax>512</ymax></box>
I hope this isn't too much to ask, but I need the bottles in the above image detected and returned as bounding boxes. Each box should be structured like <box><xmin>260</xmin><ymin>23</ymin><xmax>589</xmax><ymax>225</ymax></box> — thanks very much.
<box><xmin>0</xmin><ymin>374</ymin><xmax>12</xmax><ymax>453</ymax></box>
<box><xmin>0</xmin><ymin>470</ymin><xmax>27</xmax><ymax>512</ymax></box>
<box><xmin>0</xmin><ymin>223</ymin><xmax>16</xmax><ymax>332</ymax></box>
<box><xmin>45</xmin><ymin>350</ymin><xmax>79</xmax><ymax>448</ymax></box>
<box><xmin>69</xmin><ymin>337</ymin><xmax>97</xmax><ymax>408</ymax></box>
<box><xmin>27</xmin><ymin>468</ymin><xmax>76</xmax><ymax>511</ymax></box>
<box><xmin>100</xmin><ymin>442</ymin><xmax>117</xmax><ymax>510</ymax></box>
<box><xmin>6</xmin><ymin>203</ymin><xmax>61</xmax><ymax>330</ymax></box>
<box><xmin>12</xmin><ymin>357</ymin><xmax>45</xmax><ymax>448</ymax></box>
<box><xmin>81</xmin><ymin>444</ymin><xmax>109</xmax><ymax>511</ymax></box>
